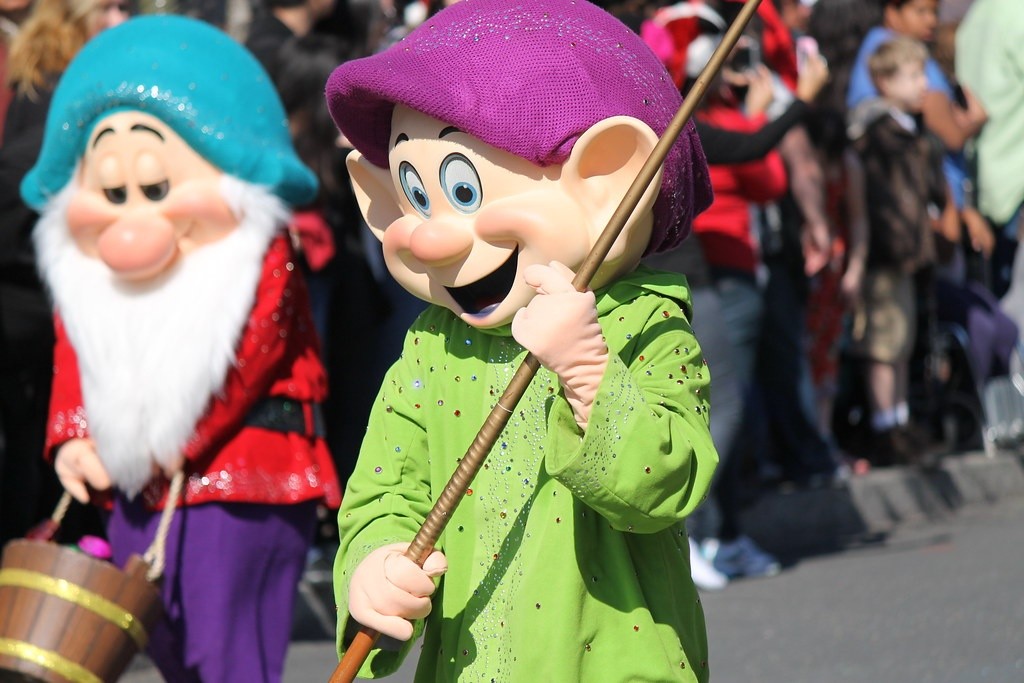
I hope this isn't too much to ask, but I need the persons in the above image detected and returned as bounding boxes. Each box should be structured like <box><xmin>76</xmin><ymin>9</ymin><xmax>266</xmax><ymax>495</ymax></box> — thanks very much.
<box><xmin>18</xmin><ymin>18</ymin><xmax>344</xmax><ymax>683</ymax></box>
<box><xmin>2</xmin><ymin>0</ymin><xmax>454</xmax><ymax>636</ymax></box>
<box><xmin>327</xmin><ymin>2</ymin><xmax>718</xmax><ymax>682</ymax></box>
<box><xmin>597</xmin><ymin>3</ymin><xmax>1023</xmax><ymax>587</ymax></box>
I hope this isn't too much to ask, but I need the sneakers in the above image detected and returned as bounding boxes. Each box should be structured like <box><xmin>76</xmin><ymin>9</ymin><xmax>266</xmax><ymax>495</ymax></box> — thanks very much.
<box><xmin>711</xmin><ymin>534</ymin><xmax>779</xmax><ymax>579</ymax></box>
<box><xmin>686</xmin><ymin>536</ymin><xmax>728</xmax><ymax>589</ymax></box>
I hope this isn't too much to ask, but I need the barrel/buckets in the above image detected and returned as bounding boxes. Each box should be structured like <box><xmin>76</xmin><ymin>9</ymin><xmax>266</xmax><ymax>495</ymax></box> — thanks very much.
<box><xmin>0</xmin><ymin>455</ymin><xmax>185</xmax><ymax>683</ymax></box>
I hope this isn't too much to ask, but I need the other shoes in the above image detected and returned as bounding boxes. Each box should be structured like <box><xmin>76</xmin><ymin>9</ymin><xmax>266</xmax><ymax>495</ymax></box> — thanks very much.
<box><xmin>847</xmin><ymin>413</ymin><xmax>948</xmax><ymax>467</ymax></box>
<box><xmin>832</xmin><ymin>528</ymin><xmax>888</xmax><ymax>550</ymax></box>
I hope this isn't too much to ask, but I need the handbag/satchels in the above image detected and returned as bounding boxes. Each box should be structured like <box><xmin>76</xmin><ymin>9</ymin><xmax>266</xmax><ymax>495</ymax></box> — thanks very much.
<box><xmin>953</xmin><ymin>242</ymin><xmax>1018</xmax><ymax>384</ymax></box>
